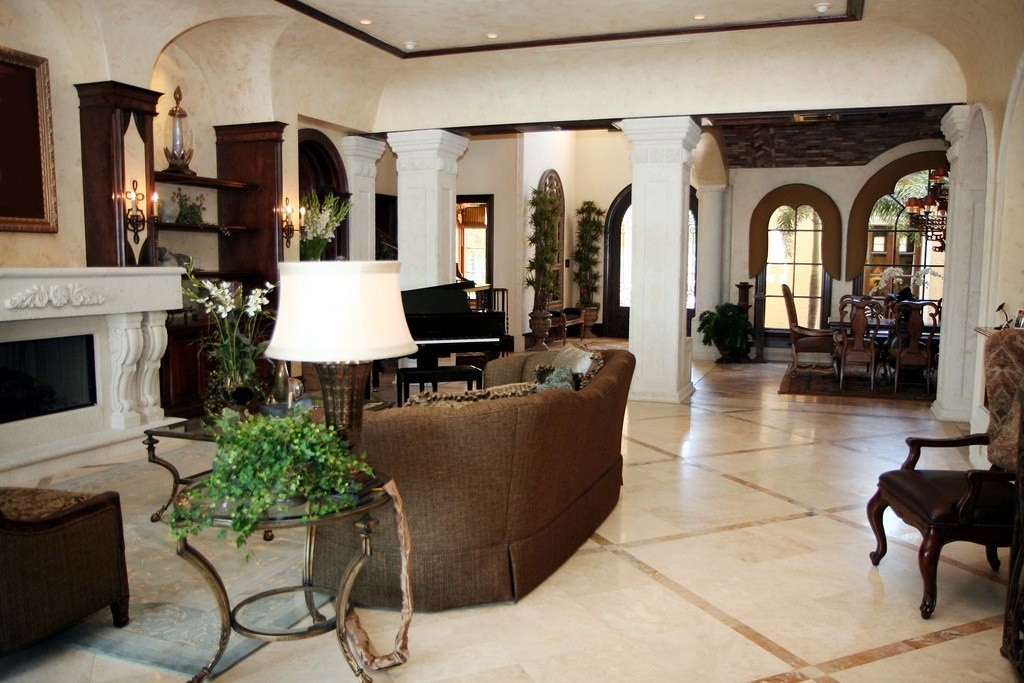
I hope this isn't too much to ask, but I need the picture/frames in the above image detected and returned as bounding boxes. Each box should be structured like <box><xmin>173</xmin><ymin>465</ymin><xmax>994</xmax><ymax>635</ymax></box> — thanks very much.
<box><xmin>1013</xmin><ymin>309</ymin><xmax>1024</xmax><ymax>330</ymax></box>
<box><xmin>0</xmin><ymin>46</ymin><xmax>59</xmax><ymax>233</ymax></box>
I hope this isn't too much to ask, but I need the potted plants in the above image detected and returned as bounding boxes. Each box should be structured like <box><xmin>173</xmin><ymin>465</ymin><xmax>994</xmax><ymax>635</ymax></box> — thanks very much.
<box><xmin>158</xmin><ymin>402</ymin><xmax>377</xmax><ymax>562</ymax></box>
<box><xmin>696</xmin><ymin>302</ymin><xmax>760</xmax><ymax>364</ymax></box>
<box><xmin>569</xmin><ymin>200</ymin><xmax>605</xmax><ymax>339</ymax></box>
<box><xmin>526</xmin><ymin>185</ymin><xmax>561</xmax><ymax>351</ymax></box>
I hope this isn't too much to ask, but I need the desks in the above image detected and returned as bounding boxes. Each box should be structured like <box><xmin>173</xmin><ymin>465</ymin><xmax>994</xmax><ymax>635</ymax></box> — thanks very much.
<box><xmin>464</xmin><ymin>284</ymin><xmax>491</xmax><ymax>312</ymax></box>
<box><xmin>975</xmin><ymin>327</ymin><xmax>996</xmax><ymax>338</ymax></box>
<box><xmin>173</xmin><ymin>470</ymin><xmax>413</xmax><ymax>683</ymax></box>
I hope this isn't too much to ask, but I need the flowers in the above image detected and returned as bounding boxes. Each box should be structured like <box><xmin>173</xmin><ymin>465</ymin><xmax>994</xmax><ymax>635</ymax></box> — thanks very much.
<box><xmin>299</xmin><ymin>186</ymin><xmax>353</xmax><ymax>261</ymax></box>
<box><xmin>868</xmin><ymin>267</ymin><xmax>943</xmax><ymax>293</ymax></box>
<box><xmin>181</xmin><ymin>254</ymin><xmax>278</xmax><ymax>414</ymax></box>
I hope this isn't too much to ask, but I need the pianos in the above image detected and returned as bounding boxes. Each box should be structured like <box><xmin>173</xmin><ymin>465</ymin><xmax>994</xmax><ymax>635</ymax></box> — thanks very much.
<box><xmin>373</xmin><ymin>282</ymin><xmax>513</xmax><ymax>393</ymax></box>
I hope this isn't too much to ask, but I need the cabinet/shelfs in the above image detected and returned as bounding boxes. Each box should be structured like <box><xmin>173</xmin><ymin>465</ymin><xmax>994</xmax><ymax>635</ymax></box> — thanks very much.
<box><xmin>153</xmin><ymin>172</ymin><xmax>274</xmax><ymax>417</ymax></box>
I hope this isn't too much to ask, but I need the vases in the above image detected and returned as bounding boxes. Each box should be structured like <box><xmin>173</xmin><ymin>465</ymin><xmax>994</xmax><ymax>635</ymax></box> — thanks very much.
<box><xmin>302</xmin><ymin>238</ymin><xmax>329</xmax><ymax>261</ymax></box>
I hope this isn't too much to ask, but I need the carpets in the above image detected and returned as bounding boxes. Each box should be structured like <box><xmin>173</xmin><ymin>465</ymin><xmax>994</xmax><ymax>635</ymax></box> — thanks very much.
<box><xmin>40</xmin><ymin>445</ymin><xmax>333</xmax><ymax>681</ymax></box>
<box><xmin>777</xmin><ymin>362</ymin><xmax>936</xmax><ymax>402</ymax></box>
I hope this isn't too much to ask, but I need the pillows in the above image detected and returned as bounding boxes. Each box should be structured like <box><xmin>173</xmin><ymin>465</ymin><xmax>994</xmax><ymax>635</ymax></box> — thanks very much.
<box><xmin>405</xmin><ymin>343</ymin><xmax>605</xmax><ymax>405</ymax></box>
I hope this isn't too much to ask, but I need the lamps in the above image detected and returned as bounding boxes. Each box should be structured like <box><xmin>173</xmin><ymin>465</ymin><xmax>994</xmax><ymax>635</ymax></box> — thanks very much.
<box><xmin>126</xmin><ymin>179</ymin><xmax>160</xmax><ymax>244</ymax></box>
<box><xmin>263</xmin><ymin>259</ymin><xmax>421</xmax><ymax>455</ymax></box>
<box><xmin>282</xmin><ymin>198</ymin><xmax>307</xmax><ymax>249</ymax></box>
<box><xmin>908</xmin><ymin>161</ymin><xmax>951</xmax><ymax>253</ymax></box>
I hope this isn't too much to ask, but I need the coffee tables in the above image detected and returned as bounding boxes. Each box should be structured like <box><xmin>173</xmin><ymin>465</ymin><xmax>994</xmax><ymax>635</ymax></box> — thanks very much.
<box><xmin>142</xmin><ymin>398</ymin><xmax>397</xmax><ymax>541</ymax></box>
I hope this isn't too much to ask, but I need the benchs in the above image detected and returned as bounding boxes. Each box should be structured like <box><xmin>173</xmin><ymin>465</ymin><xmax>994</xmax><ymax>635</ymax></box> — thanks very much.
<box><xmin>312</xmin><ymin>348</ymin><xmax>634</xmax><ymax>615</ymax></box>
<box><xmin>548</xmin><ymin>307</ymin><xmax>585</xmax><ymax>346</ymax></box>
<box><xmin>0</xmin><ymin>484</ymin><xmax>129</xmax><ymax>661</ymax></box>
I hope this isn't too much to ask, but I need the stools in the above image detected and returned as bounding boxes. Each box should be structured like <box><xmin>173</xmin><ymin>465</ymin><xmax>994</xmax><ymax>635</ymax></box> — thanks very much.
<box><xmin>397</xmin><ymin>365</ymin><xmax>484</xmax><ymax>408</ymax></box>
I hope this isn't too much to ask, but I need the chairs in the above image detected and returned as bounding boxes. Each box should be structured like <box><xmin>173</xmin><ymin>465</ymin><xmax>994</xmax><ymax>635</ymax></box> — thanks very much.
<box><xmin>866</xmin><ymin>330</ymin><xmax>1024</xmax><ymax>620</ymax></box>
<box><xmin>781</xmin><ymin>284</ymin><xmax>943</xmax><ymax>395</ymax></box>
